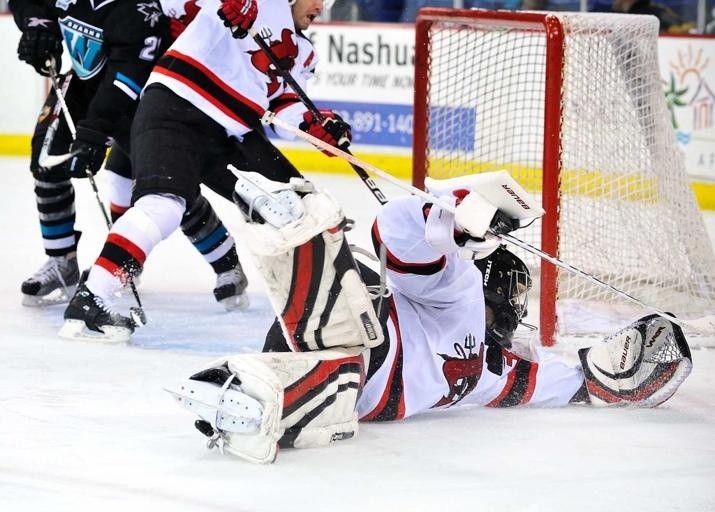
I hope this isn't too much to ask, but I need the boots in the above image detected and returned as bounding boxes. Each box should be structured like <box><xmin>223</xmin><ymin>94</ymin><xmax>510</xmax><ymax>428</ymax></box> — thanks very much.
<box><xmin>21</xmin><ymin>228</ymin><xmax>84</xmax><ymax>297</ymax></box>
<box><xmin>187</xmin><ymin>362</ymin><xmax>243</xmax><ymax>440</ymax></box>
<box><xmin>212</xmin><ymin>259</ymin><xmax>248</xmax><ymax>301</ymax></box>
<box><xmin>231</xmin><ymin>190</ymin><xmax>266</xmax><ymax>224</ymax></box>
<box><xmin>62</xmin><ymin>267</ymin><xmax>136</xmax><ymax>334</ymax></box>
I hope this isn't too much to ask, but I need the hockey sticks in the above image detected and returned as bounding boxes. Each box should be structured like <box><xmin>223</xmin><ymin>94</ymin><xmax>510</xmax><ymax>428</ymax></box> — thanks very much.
<box><xmin>45</xmin><ymin>57</ymin><xmax>148</xmax><ymax>326</ymax></box>
<box><xmin>261</xmin><ymin>110</ymin><xmax>715</xmax><ymax>336</ymax></box>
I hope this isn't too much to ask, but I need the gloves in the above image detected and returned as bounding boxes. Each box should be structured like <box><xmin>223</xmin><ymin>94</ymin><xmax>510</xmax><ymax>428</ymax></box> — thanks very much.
<box><xmin>299</xmin><ymin>107</ymin><xmax>353</xmax><ymax>159</ymax></box>
<box><xmin>67</xmin><ymin>120</ymin><xmax>111</xmax><ymax>180</ymax></box>
<box><xmin>17</xmin><ymin>8</ymin><xmax>63</xmax><ymax>78</ymax></box>
<box><xmin>217</xmin><ymin>0</ymin><xmax>259</xmax><ymax>40</ymax></box>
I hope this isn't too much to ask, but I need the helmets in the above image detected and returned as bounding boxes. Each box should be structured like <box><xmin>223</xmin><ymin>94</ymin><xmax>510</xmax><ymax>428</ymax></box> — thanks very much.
<box><xmin>475</xmin><ymin>247</ymin><xmax>533</xmax><ymax>346</ymax></box>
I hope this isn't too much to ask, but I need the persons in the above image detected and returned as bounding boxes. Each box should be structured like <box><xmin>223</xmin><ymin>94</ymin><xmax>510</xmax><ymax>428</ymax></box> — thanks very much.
<box><xmin>183</xmin><ymin>168</ymin><xmax>694</xmax><ymax>463</ymax></box>
<box><xmin>61</xmin><ymin>0</ymin><xmax>351</xmax><ymax>331</ymax></box>
<box><xmin>9</xmin><ymin>0</ymin><xmax>247</xmax><ymax>299</ymax></box>
